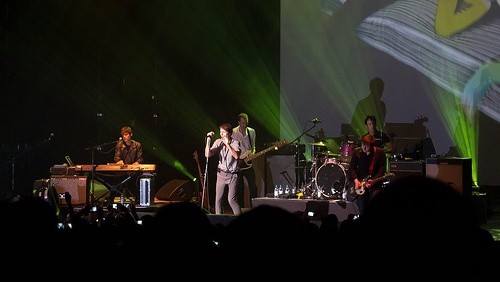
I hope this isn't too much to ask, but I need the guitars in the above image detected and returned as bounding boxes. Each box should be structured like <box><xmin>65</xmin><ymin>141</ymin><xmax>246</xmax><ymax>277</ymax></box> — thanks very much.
<box><xmin>194</xmin><ymin>151</ymin><xmax>209</xmax><ymax>213</ymax></box>
<box><xmin>239</xmin><ymin>138</ymin><xmax>287</xmax><ymax>170</ymax></box>
<box><xmin>348</xmin><ymin>172</ymin><xmax>395</xmax><ymax>202</ymax></box>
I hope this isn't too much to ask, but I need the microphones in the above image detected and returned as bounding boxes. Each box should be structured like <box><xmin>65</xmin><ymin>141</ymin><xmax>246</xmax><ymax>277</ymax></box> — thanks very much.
<box><xmin>118</xmin><ymin>137</ymin><xmax>122</xmax><ymax>145</ymax></box>
<box><xmin>48</xmin><ymin>133</ymin><xmax>54</xmax><ymax>141</ymax></box>
<box><xmin>280</xmin><ymin>171</ymin><xmax>287</xmax><ymax>174</ymax></box>
<box><xmin>310</xmin><ymin>118</ymin><xmax>322</xmax><ymax>124</ymax></box>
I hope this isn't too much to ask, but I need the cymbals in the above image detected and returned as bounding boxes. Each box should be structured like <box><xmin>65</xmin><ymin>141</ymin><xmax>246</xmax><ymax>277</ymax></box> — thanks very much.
<box><xmin>306</xmin><ymin>142</ymin><xmax>331</xmax><ymax>146</ymax></box>
<box><xmin>305</xmin><ymin>133</ymin><xmax>328</xmax><ymax>144</ymax></box>
<box><xmin>315</xmin><ymin>151</ymin><xmax>340</xmax><ymax>157</ymax></box>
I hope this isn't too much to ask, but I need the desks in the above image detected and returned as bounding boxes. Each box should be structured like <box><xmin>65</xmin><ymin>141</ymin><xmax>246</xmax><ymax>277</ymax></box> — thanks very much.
<box><xmin>252</xmin><ymin>197</ymin><xmax>360</xmax><ymax>222</ymax></box>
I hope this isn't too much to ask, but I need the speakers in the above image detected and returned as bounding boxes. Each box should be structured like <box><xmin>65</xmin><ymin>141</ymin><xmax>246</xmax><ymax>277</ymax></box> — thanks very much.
<box><xmin>154</xmin><ymin>180</ymin><xmax>194</xmax><ymax>201</ymax></box>
<box><xmin>389</xmin><ymin>159</ymin><xmax>423</xmax><ymax>182</ymax></box>
<box><xmin>266</xmin><ymin>155</ymin><xmax>297</xmax><ymax>198</ymax></box>
<box><xmin>89</xmin><ymin>180</ymin><xmax>110</xmax><ymax>205</ymax></box>
<box><xmin>236</xmin><ymin>155</ymin><xmax>266</xmax><ymax>208</ymax></box>
<box><xmin>425</xmin><ymin>156</ymin><xmax>472</xmax><ymax>197</ymax></box>
<box><xmin>50</xmin><ymin>176</ymin><xmax>88</xmax><ymax>205</ymax></box>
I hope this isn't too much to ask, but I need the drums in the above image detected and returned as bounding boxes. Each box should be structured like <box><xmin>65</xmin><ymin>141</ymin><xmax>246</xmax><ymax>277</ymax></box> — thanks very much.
<box><xmin>312</xmin><ymin>157</ymin><xmax>338</xmax><ymax>179</ymax></box>
<box><xmin>288</xmin><ymin>143</ymin><xmax>306</xmax><ymax>153</ymax></box>
<box><xmin>338</xmin><ymin>140</ymin><xmax>356</xmax><ymax>162</ymax></box>
<box><xmin>314</xmin><ymin>161</ymin><xmax>350</xmax><ymax>198</ymax></box>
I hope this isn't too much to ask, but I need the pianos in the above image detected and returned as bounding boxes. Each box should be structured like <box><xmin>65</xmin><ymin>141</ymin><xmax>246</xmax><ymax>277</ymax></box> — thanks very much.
<box><xmin>75</xmin><ymin>161</ymin><xmax>158</xmax><ymax>206</ymax></box>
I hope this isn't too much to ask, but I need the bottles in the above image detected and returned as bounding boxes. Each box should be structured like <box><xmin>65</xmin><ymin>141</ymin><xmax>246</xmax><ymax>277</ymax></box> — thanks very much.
<box><xmin>279</xmin><ymin>185</ymin><xmax>283</xmax><ymax>194</ymax></box>
<box><xmin>274</xmin><ymin>185</ymin><xmax>278</xmax><ymax>198</ymax></box>
<box><xmin>292</xmin><ymin>186</ymin><xmax>296</xmax><ymax>194</ymax></box>
<box><xmin>284</xmin><ymin>185</ymin><xmax>289</xmax><ymax>194</ymax></box>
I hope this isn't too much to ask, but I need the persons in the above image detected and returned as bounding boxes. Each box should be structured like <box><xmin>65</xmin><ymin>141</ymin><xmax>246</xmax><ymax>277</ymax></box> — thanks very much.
<box><xmin>205</xmin><ymin>123</ymin><xmax>241</xmax><ymax>214</ymax></box>
<box><xmin>365</xmin><ymin>116</ymin><xmax>393</xmax><ymax>152</ymax></box>
<box><xmin>231</xmin><ymin>113</ymin><xmax>258</xmax><ymax>208</ymax></box>
<box><xmin>107</xmin><ymin>125</ymin><xmax>143</xmax><ymax>217</ymax></box>
<box><xmin>346</xmin><ymin>133</ymin><xmax>386</xmax><ymax>198</ymax></box>
<box><xmin>0</xmin><ymin>172</ymin><xmax>500</xmax><ymax>282</ymax></box>
